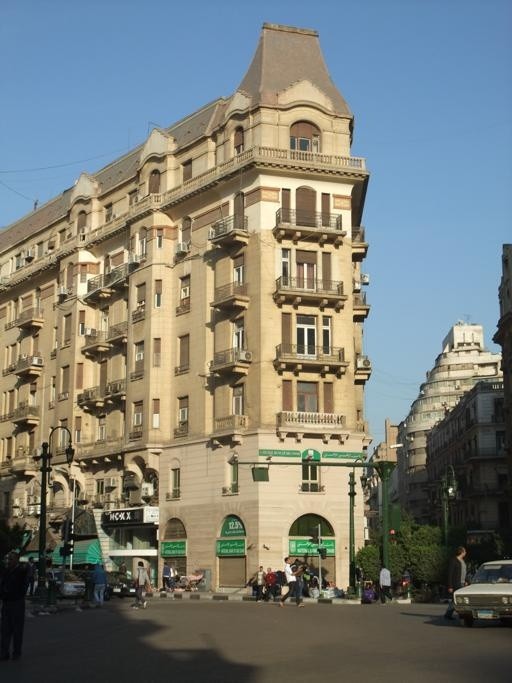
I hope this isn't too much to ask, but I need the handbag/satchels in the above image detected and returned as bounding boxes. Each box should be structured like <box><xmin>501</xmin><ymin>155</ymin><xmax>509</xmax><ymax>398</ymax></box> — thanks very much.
<box><xmin>290</xmin><ymin>559</ymin><xmax>309</xmax><ymax>576</ymax></box>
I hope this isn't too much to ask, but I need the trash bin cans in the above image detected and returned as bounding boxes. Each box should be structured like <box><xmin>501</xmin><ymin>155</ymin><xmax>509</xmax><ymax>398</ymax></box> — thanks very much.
<box><xmin>361</xmin><ymin>581</ymin><xmax>374</xmax><ymax>604</ymax></box>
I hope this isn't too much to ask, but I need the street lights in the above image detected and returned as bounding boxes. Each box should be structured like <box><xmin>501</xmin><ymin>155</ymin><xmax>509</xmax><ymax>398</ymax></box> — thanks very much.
<box><xmin>37</xmin><ymin>424</ymin><xmax>76</xmax><ymax>600</ymax></box>
<box><xmin>437</xmin><ymin>464</ymin><xmax>462</xmax><ymax>555</ymax></box>
<box><xmin>347</xmin><ymin>457</ymin><xmax>370</xmax><ymax>589</ymax></box>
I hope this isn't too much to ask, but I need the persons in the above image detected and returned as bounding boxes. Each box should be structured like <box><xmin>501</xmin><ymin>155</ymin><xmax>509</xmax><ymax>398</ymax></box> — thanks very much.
<box><xmin>129</xmin><ymin>560</ymin><xmax>150</xmax><ymax>609</ymax></box>
<box><xmin>0</xmin><ymin>551</ymin><xmax>32</xmax><ymax>659</ymax></box>
<box><xmin>444</xmin><ymin>546</ymin><xmax>469</xmax><ymax>619</ymax></box>
<box><xmin>162</xmin><ymin>561</ymin><xmax>170</xmax><ymax>590</ymax></box>
<box><xmin>378</xmin><ymin>563</ymin><xmax>393</xmax><ymax>606</ymax></box>
<box><xmin>0</xmin><ymin>551</ymin><xmax>54</xmax><ymax>596</ymax></box>
<box><xmin>77</xmin><ymin>560</ymin><xmax>128</xmax><ymax>608</ymax></box>
<box><xmin>169</xmin><ymin>564</ymin><xmax>178</xmax><ymax>591</ymax></box>
<box><xmin>247</xmin><ymin>556</ymin><xmax>320</xmax><ymax>608</ymax></box>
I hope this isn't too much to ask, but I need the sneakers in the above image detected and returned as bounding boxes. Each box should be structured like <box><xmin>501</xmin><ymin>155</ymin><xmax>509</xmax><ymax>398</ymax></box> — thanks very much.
<box><xmin>445</xmin><ymin>615</ymin><xmax>474</xmax><ymax>627</ymax></box>
<box><xmin>257</xmin><ymin>597</ymin><xmax>305</xmax><ymax>608</ymax></box>
<box><xmin>380</xmin><ymin>597</ymin><xmax>396</xmax><ymax>607</ymax></box>
<box><xmin>0</xmin><ymin>652</ymin><xmax>25</xmax><ymax>660</ymax></box>
<box><xmin>97</xmin><ymin>600</ymin><xmax>148</xmax><ymax>610</ymax></box>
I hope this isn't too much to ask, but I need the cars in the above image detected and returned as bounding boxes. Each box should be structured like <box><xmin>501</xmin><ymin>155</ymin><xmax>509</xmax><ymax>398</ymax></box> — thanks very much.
<box><xmin>36</xmin><ymin>567</ymin><xmax>138</xmax><ymax>600</ymax></box>
<box><xmin>453</xmin><ymin>558</ymin><xmax>512</xmax><ymax>626</ymax></box>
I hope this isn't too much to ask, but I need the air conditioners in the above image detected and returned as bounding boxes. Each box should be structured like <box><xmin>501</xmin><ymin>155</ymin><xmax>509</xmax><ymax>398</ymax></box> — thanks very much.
<box><xmin>84</xmin><ymin>326</ymin><xmax>97</xmax><ymax>337</ymax></box>
<box><xmin>57</xmin><ymin>287</ymin><xmax>67</xmax><ymax>296</ymax></box>
<box><xmin>238</xmin><ymin>351</ymin><xmax>252</xmax><ymax>363</ymax></box>
<box><xmin>213</xmin><ymin>221</ymin><xmax>227</xmax><ymax>235</ymax></box>
<box><xmin>357</xmin><ymin>355</ymin><xmax>371</xmax><ymax>369</ymax></box>
<box><xmin>361</xmin><ymin>272</ymin><xmax>369</xmax><ymax>284</ymax></box>
<box><xmin>32</xmin><ymin>356</ymin><xmax>44</xmax><ymax>366</ymax></box>
<box><xmin>128</xmin><ymin>252</ymin><xmax>140</xmax><ymax>265</ymax></box>
<box><xmin>174</xmin><ymin>242</ymin><xmax>187</xmax><ymax>255</ymax></box>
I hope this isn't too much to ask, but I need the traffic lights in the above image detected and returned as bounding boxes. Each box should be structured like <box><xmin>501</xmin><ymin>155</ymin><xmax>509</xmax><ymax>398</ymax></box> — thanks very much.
<box><xmin>64</xmin><ymin>543</ymin><xmax>74</xmax><ymax>556</ymax></box>
<box><xmin>62</xmin><ymin>519</ymin><xmax>74</xmax><ymax>541</ymax></box>
<box><xmin>387</xmin><ymin>528</ymin><xmax>399</xmax><ymax>545</ymax></box>
<box><xmin>317</xmin><ymin>548</ymin><xmax>326</xmax><ymax>560</ymax></box>
<box><xmin>309</xmin><ymin>527</ymin><xmax>318</xmax><ymax>542</ymax></box>
